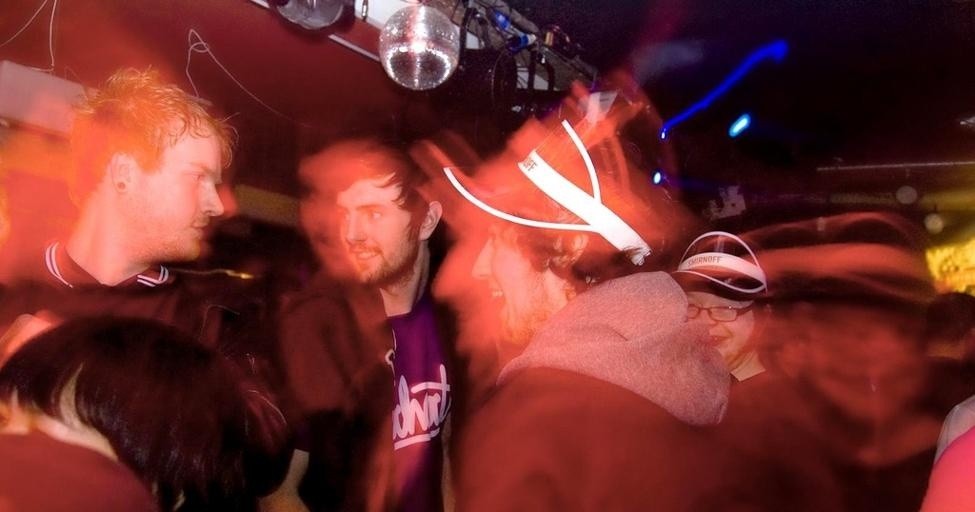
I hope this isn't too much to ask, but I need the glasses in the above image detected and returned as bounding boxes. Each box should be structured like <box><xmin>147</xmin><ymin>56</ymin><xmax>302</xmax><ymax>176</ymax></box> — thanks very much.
<box><xmin>687</xmin><ymin>301</ymin><xmax>759</xmax><ymax>322</ymax></box>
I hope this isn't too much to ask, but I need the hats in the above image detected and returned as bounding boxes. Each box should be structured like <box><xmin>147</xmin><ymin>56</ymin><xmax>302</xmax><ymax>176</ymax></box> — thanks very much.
<box><xmin>666</xmin><ymin>229</ymin><xmax>771</xmax><ymax>298</ymax></box>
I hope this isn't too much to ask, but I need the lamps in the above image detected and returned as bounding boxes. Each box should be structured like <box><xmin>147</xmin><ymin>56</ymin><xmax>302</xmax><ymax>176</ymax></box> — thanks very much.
<box><xmin>268</xmin><ymin>1</ymin><xmax>560</xmax><ymax>128</ymax></box>
